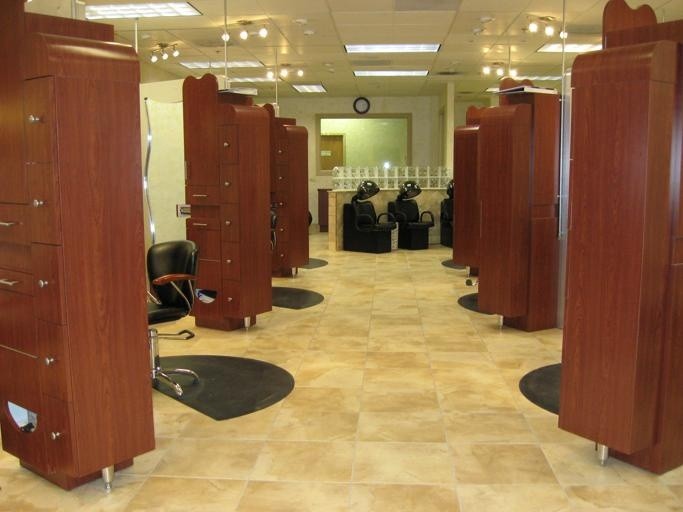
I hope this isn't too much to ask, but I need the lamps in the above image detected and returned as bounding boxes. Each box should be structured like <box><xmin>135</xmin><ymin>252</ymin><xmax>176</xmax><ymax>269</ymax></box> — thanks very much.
<box><xmin>525</xmin><ymin>15</ymin><xmax>568</xmax><ymax>40</ymax></box>
<box><xmin>481</xmin><ymin>62</ymin><xmax>518</xmax><ymax>77</ymax></box>
<box><xmin>220</xmin><ymin>19</ymin><xmax>267</xmax><ymax>41</ymax></box>
<box><xmin>150</xmin><ymin>41</ymin><xmax>179</xmax><ymax>63</ymax></box>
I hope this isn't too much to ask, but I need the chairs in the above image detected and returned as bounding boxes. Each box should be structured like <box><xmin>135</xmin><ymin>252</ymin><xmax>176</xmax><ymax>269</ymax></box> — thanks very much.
<box><xmin>440</xmin><ymin>199</ymin><xmax>452</xmax><ymax>248</ymax></box>
<box><xmin>146</xmin><ymin>240</ymin><xmax>200</xmax><ymax>402</ymax></box>
<box><xmin>387</xmin><ymin>199</ymin><xmax>434</xmax><ymax>250</ymax></box>
<box><xmin>343</xmin><ymin>201</ymin><xmax>396</xmax><ymax>253</ymax></box>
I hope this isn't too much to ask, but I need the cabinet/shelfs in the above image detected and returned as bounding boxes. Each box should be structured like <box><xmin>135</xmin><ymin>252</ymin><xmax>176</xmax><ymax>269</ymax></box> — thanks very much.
<box><xmin>452</xmin><ymin>105</ymin><xmax>487</xmax><ymax>276</ymax></box>
<box><xmin>556</xmin><ymin>0</ymin><xmax>683</xmax><ymax>477</ymax></box>
<box><xmin>182</xmin><ymin>73</ymin><xmax>274</xmax><ymax>333</ymax></box>
<box><xmin>474</xmin><ymin>77</ymin><xmax>561</xmax><ymax>333</ymax></box>
<box><xmin>263</xmin><ymin>102</ymin><xmax>310</xmax><ymax>278</ymax></box>
<box><xmin>0</xmin><ymin>0</ymin><xmax>156</xmax><ymax>496</ymax></box>
